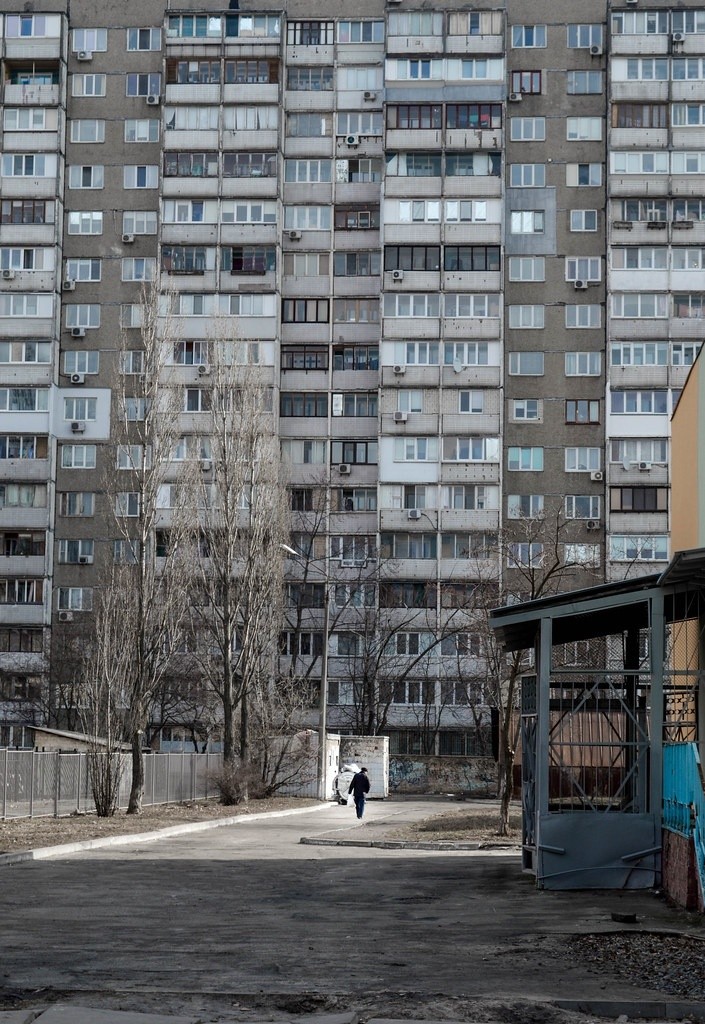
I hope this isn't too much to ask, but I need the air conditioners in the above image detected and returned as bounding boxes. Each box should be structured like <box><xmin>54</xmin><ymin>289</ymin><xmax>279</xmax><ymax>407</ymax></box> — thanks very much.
<box><xmin>59</xmin><ymin>612</ymin><xmax>74</xmax><ymax>621</ymax></box>
<box><xmin>71</xmin><ymin>327</ymin><xmax>86</xmax><ymax>336</ymax></box>
<box><xmin>573</xmin><ymin>280</ymin><xmax>588</xmax><ymax>288</ymax></box>
<box><xmin>590</xmin><ymin>472</ymin><xmax>603</xmax><ymax>480</ymax></box>
<box><xmin>71</xmin><ymin>374</ymin><xmax>86</xmax><ymax>382</ymax></box>
<box><xmin>586</xmin><ymin>520</ymin><xmax>601</xmax><ymax>529</ymax></box>
<box><xmin>673</xmin><ymin>33</ymin><xmax>685</xmax><ymax>41</ymax></box>
<box><xmin>77</xmin><ymin>50</ymin><xmax>93</xmax><ymax>60</ymax></box>
<box><xmin>71</xmin><ymin>421</ymin><xmax>84</xmax><ymax>432</ymax></box>
<box><xmin>3</xmin><ymin>269</ymin><xmax>14</xmax><ymax>279</ymax></box>
<box><xmin>196</xmin><ymin>269</ymin><xmax>420</xmax><ymax>522</ymax></box>
<box><xmin>639</xmin><ymin>462</ymin><xmax>651</xmax><ymax>470</ymax></box>
<box><xmin>122</xmin><ymin>233</ymin><xmax>134</xmax><ymax>242</ymax></box>
<box><xmin>345</xmin><ymin>135</ymin><xmax>360</xmax><ymax>145</ymax></box>
<box><xmin>363</xmin><ymin>91</ymin><xmax>375</xmax><ymax>99</ymax></box>
<box><xmin>625</xmin><ymin>0</ymin><xmax>638</xmax><ymax>3</ymax></box>
<box><xmin>289</xmin><ymin>230</ymin><xmax>303</xmax><ymax>238</ymax></box>
<box><xmin>147</xmin><ymin>94</ymin><xmax>159</xmax><ymax>104</ymax></box>
<box><xmin>590</xmin><ymin>46</ymin><xmax>603</xmax><ymax>55</ymax></box>
<box><xmin>63</xmin><ymin>280</ymin><xmax>76</xmax><ymax>290</ymax></box>
<box><xmin>78</xmin><ymin>555</ymin><xmax>92</xmax><ymax>563</ymax></box>
<box><xmin>509</xmin><ymin>93</ymin><xmax>522</xmax><ymax>101</ymax></box>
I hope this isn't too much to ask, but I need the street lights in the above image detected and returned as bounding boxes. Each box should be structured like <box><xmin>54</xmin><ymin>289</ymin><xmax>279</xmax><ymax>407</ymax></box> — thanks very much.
<box><xmin>278</xmin><ymin>541</ymin><xmax>330</xmax><ymax>802</ymax></box>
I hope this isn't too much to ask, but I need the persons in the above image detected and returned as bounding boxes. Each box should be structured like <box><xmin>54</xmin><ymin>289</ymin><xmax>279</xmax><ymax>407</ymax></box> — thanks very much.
<box><xmin>347</xmin><ymin>767</ymin><xmax>371</xmax><ymax>819</ymax></box>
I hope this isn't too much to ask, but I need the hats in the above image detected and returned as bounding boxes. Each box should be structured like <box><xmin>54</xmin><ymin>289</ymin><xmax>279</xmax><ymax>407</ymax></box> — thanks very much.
<box><xmin>361</xmin><ymin>768</ymin><xmax>368</xmax><ymax>771</ymax></box>
